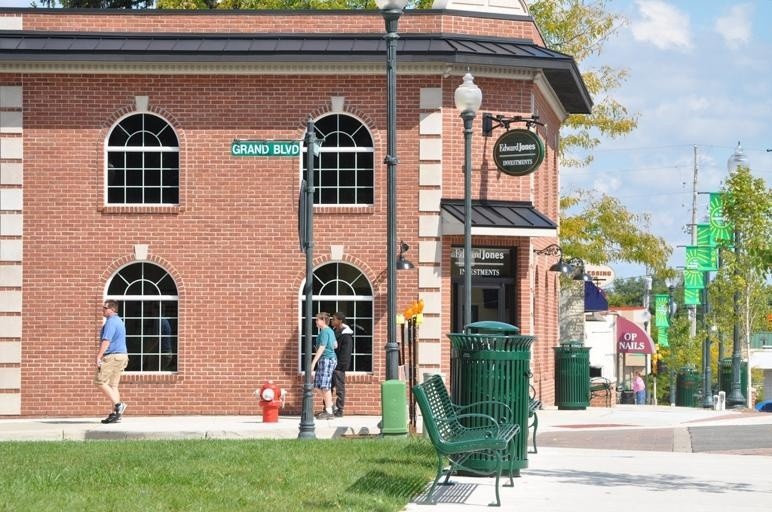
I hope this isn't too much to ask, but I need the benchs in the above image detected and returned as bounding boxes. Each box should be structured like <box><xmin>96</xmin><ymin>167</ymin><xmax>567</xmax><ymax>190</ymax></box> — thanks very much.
<box><xmin>528</xmin><ymin>383</ymin><xmax>544</xmax><ymax>454</ymax></box>
<box><xmin>412</xmin><ymin>373</ymin><xmax>521</xmax><ymax>507</ymax></box>
<box><xmin>589</xmin><ymin>374</ymin><xmax>614</xmax><ymax>408</ymax></box>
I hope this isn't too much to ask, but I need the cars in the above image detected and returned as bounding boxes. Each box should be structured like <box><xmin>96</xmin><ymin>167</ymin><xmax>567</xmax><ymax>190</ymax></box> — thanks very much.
<box><xmin>755</xmin><ymin>400</ymin><xmax>772</xmax><ymax>412</ymax></box>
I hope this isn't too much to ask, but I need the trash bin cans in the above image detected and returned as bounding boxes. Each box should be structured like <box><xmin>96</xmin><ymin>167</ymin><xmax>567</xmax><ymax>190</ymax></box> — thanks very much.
<box><xmin>551</xmin><ymin>341</ymin><xmax>592</xmax><ymax>410</ymax></box>
<box><xmin>675</xmin><ymin>367</ymin><xmax>699</xmax><ymax>407</ymax></box>
<box><xmin>446</xmin><ymin>321</ymin><xmax>537</xmax><ymax>477</ymax></box>
<box><xmin>721</xmin><ymin>357</ymin><xmax>748</xmax><ymax>409</ymax></box>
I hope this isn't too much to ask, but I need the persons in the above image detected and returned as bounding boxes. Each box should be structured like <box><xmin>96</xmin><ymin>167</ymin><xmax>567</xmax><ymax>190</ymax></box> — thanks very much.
<box><xmin>93</xmin><ymin>300</ymin><xmax>129</xmax><ymax>423</ymax></box>
<box><xmin>148</xmin><ymin>304</ymin><xmax>175</xmax><ymax>370</ymax></box>
<box><xmin>330</xmin><ymin>311</ymin><xmax>353</xmax><ymax>416</ymax></box>
<box><xmin>311</xmin><ymin>312</ymin><xmax>339</xmax><ymax>419</ymax></box>
<box><xmin>633</xmin><ymin>371</ymin><xmax>645</xmax><ymax>405</ymax></box>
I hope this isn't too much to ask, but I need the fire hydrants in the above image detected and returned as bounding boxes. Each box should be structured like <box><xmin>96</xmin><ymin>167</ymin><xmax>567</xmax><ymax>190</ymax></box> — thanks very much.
<box><xmin>256</xmin><ymin>381</ymin><xmax>285</xmax><ymax>423</ymax></box>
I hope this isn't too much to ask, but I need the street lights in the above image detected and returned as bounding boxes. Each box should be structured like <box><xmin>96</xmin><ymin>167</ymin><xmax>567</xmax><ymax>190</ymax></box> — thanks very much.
<box><xmin>374</xmin><ymin>1</ymin><xmax>418</xmax><ymax>377</ymax></box>
<box><xmin>664</xmin><ymin>274</ymin><xmax>681</xmax><ymax>406</ymax></box>
<box><xmin>453</xmin><ymin>73</ymin><xmax>484</xmax><ymax>339</ymax></box>
<box><xmin>724</xmin><ymin>139</ymin><xmax>746</xmax><ymax>410</ymax></box>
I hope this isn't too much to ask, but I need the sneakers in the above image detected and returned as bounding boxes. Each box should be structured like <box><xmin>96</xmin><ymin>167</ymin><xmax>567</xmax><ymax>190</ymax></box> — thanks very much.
<box><xmin>102</xmin><ymin>413</ymin><xmax>121</xmax><ymax>423</ymax></box>
<box><xmin>117</xmin><ymin>403</ymin><xmax>128</xmax><ymax>418</ymax></box>
<box><xmin>315</xmin><ymin>410</ymin><xmax>343</xmax><ymax>420</ymax></box>
